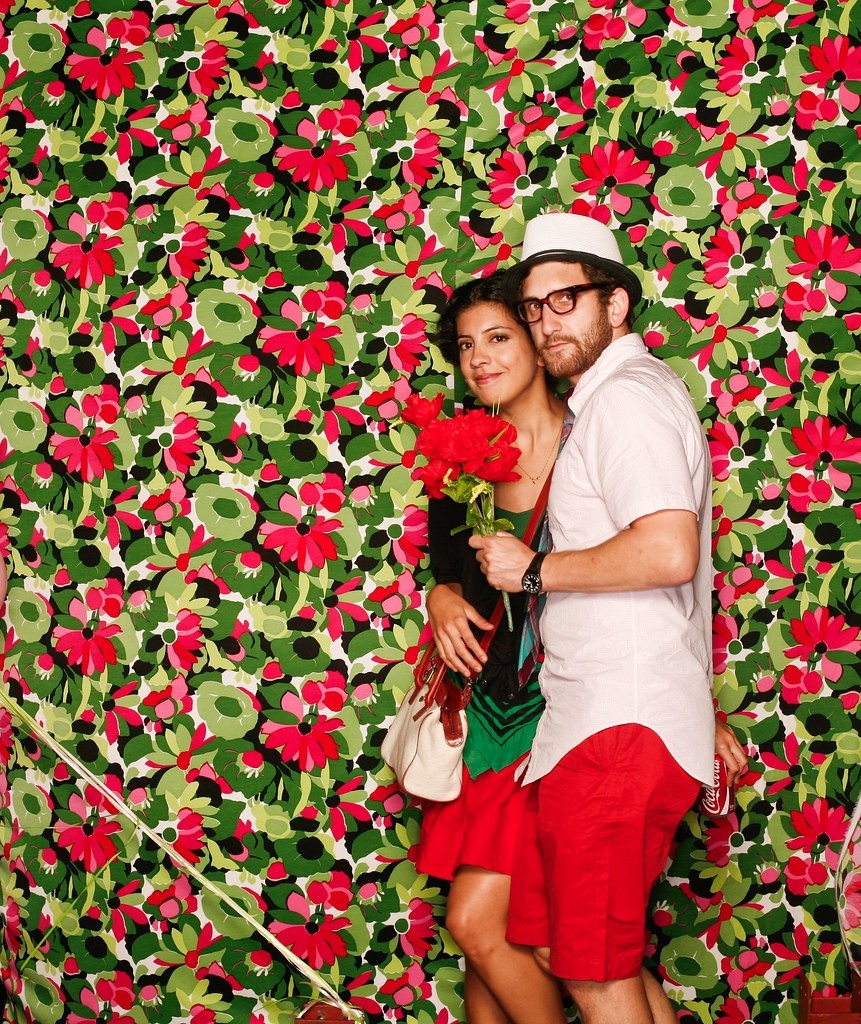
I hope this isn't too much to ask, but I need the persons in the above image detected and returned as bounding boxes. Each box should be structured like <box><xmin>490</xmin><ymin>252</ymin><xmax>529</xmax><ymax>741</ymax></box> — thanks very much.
<box><xmin>417</xmin><ymin>270</ymin><xmax>748</xmax><ymax>1024</ymax></box>
<box><xmin>470</xmin><ymin>212</ymin><xmax>718</xmax><ymax>1024</ymax></box>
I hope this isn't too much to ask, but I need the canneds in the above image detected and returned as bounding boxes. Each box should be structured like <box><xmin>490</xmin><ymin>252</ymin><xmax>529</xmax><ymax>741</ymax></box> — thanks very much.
<box><xmin>697</xmin><ymin>753</ymin><xmax>735</xmax><ymax>817</ymax></box>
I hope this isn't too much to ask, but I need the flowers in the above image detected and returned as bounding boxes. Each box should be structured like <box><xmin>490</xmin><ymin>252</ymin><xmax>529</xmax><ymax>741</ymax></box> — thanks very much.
<box><xmin>401</xmin><ymin>391</ymin><xmax>523</xmax><ymax>632</ymax></box>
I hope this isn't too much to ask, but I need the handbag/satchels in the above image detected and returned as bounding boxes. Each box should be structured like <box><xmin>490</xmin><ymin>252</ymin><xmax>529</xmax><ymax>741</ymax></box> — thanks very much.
<box><xmin>381</xmin><ymin>659</ymin><xmax>469</xmax><ymax>803</ymax></box>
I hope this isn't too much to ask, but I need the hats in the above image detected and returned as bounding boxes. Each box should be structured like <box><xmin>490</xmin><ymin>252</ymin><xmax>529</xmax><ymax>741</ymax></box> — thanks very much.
<box><xmin>503</xmin><ymin>213</ymin><xmax>642</xmax><ymax>311</ymax></box>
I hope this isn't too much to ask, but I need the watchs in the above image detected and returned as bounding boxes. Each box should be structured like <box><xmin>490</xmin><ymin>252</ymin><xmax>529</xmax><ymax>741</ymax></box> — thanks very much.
<box><xmin>521</xmin><ymin>551</ymin><xmax>547</xmax><ymax>595</ymax></box>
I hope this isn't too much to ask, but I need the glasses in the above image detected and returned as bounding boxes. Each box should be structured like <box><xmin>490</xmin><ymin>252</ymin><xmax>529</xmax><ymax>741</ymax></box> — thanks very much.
<box><xmin>517</xmin><ymin>282</ymin><xmax>611</xmax><ymax>324</ymax></box>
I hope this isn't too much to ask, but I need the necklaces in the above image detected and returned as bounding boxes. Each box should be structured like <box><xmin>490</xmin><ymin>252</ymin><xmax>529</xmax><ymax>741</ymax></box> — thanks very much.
<box><xmin>517</xmin><ymin>428</ymin><xmax>560</xmax><ymax>483</ymax></box>
